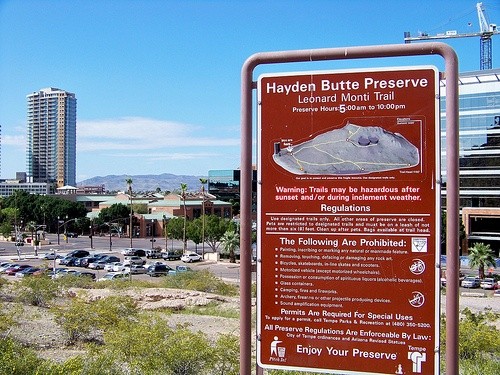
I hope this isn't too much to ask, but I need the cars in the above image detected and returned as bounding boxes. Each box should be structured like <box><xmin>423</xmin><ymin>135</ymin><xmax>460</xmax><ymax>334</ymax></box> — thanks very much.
<box><xmin>97</xmin><ymin>271</ymin><xmax>132</xmax><ymax>282</ymax></box>
<box><xmin>64</xmin><ymin>232</ymin><xmax>79</xmax><ymax>238</ymax></box>
<box><xmin>460</xmin><ymin>275</ymin><xmax>497</xmax><ymax>289</ymax></box>
<box><xmin>59</xmin><ymin>250</ymin><xmax>193</xmax><ymax>277</ymax></box>
<box><xmin>45</xmin><ymin>252</ymin><xmax>61</xmax><ymax>260</ymax></box>
<box><xmin>180</xmin><ymin>251</ymin><xmax>203</xmax><ymax>263</ymax></box>
<box><xmin>0</xmin><ymin>262</ymin><xmax>96</xmax><ymax>281</ymax></box>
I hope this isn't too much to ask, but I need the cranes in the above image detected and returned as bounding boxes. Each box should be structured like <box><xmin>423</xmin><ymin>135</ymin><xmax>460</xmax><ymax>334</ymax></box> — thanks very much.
<box><xmin>404</xmin><ymin>2</ymin><xmax>500</xmax><ymax>70</ymax></box>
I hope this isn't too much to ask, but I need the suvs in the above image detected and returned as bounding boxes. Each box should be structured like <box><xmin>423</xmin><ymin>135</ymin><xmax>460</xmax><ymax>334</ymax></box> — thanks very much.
<box><xmin>123</xmin><ymin>248</ymin><xmax>182</xmax><ymax>259</ymax></box>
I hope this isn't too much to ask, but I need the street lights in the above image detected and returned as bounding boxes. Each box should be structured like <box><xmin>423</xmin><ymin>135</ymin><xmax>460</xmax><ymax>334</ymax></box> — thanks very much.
<box><xmin>151</xmin><ymin>218</ymin><xmax>154</xmax><ymax>248</ymax></box>
<box><xmin>57</xmin><ymin>215</ymin><xmax>60</xmax><ymax>245</ymax></box>
<box><xmin>89</xmin><ymin>217</ymin><xmax>94</xmax><ymax>248</ymax></box>
<box><xmin>21</xmin><ymin>220</ymin><xmax>24</xmax><ymax>231</ymax></box>
